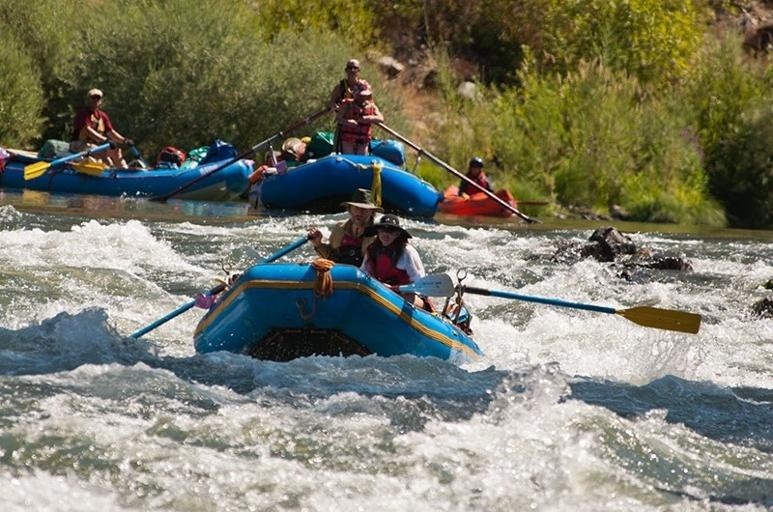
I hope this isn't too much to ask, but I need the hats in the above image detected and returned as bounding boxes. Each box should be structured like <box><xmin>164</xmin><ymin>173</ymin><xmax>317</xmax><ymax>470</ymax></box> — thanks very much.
<box><xmin>339</xmin><ymin>187</ymin><xmax>387</xmax><ymax>215</ymax></box>
<box><xmin>362</xmin><ymin>212</ymin><xmax>414</xmax><ymax>239</ymax></box>
<box><xmin>348</xmin><ymin>79</ymin><xmax>374</xmax><ymax>97</ymax></box>
<box><xmin>85</xmin><ymin>88</ymin><xmax>105</xmax><ymax>100</ymax></box>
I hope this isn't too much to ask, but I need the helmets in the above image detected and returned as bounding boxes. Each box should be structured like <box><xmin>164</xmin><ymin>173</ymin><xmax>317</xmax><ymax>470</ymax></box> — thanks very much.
<box><xmin>468</xmin><ymin>156</ymin><xmax>485</xmax><ymax>169</ymax></box>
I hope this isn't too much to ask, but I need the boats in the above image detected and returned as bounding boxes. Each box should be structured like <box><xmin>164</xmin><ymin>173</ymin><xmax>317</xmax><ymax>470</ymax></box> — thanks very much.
<box><xmin>245</xmin><ymin>154</ymin><xmax>443</xmax><ymax>219</ymax></box>
<box><xmin>440</xmin><ymin>184</ymin><xmax>518</xmax><ymax>217</ymax></box>
<box><xmin>0</xmin><ymin>152</ymin><xmax>253</xmax><ymax>202</ymax></box>
<box><xmin>189</xmin><ymin>260</ymin><xmax>487</xmax><ymax>368</ymax></box>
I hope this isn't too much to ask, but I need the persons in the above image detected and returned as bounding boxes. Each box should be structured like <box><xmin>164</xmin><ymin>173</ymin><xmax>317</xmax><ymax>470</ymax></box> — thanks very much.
<box><xmin>330</xmin><ymin>59</ymin><xmax>371</xmax><ymax>155</ymax></box>
<box><xmin>335</xmin><ymin>78</ymin><xmax>384</xmax><ymax>155</ymax></box>
<box><xmin>305</xmin><ymin>190</ymin><xmax>375</xmax><ymax>269</ymax></box>
<box><xmin>67</xmin><ymin>88</ymin><xmax>127</xmax><ymax>172</ymax></box>
<box><xmin>356</xmin><ymin>214</ymin><xmax>425</xmax><ymax>308</ymax></box>
<box><xmin>456</xmin><ymin>157</ymin><xmax>493</xmax><ymax>201</ymax></box>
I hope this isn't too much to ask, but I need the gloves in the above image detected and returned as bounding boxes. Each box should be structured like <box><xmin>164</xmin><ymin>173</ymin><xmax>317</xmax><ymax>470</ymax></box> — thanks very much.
<box><xmin>125</xmin><ymin>138</ymin><xmax>135</xmax><ymax>146</ymax></box>
<box><xmin>102</xmin><ymin>137</ymin><xmax>118</xmax><ymax>150</ymax></box>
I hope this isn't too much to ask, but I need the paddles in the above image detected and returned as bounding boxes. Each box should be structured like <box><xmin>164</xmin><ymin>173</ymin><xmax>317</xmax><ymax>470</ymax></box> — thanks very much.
<box><xmin>17</xmin><ymin>142</ymin><xmax>105</xmax><ymax>180</ymax></box>
<box><xmin>379</xmin><ymin>123</ymin><xmax>543</xmax><ymax>227</ymax></box>
<box><xmin>390</xmin><ymin>274</ymin><xmax>455</xmax><ymax>299</ymax></box>
<box><xmin>148</xmin><ymin>107</ymin><xmax>332</xmax><ymax>204</ymax></box>
<box><xmin>458</xmin><ymin>285</ymin><xmax>700</xmax><ymax>333</ymax></box>
